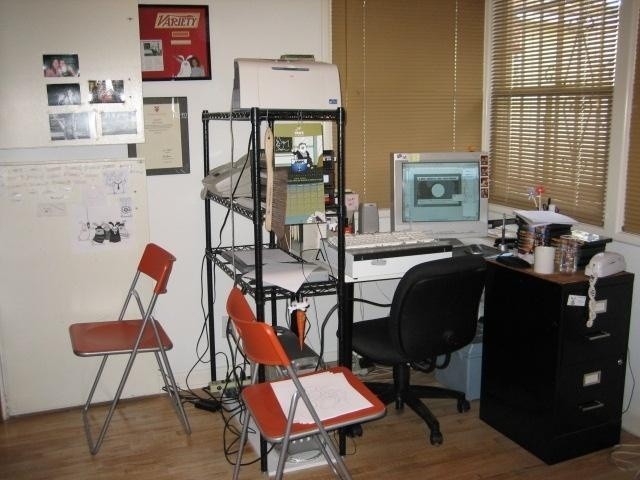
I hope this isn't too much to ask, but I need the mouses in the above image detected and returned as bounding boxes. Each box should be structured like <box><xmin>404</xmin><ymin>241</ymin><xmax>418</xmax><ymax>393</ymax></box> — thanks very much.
<box><xmin>469</xmin><ymin>244</ymin><xmax>484</xmax><ymax>255</ymax></box>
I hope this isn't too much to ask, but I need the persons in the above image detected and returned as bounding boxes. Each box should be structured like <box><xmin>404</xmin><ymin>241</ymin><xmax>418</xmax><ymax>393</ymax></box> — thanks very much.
<box><xmin>45</xmin><ymin>59</ymin><xmax>60</xmax><ymax>76</ymax></box>
<box><xmin>57</xmin><ymin>60</ymin><xmax>74</xmax><ymax>76</ymax></box>
<box><xmin>61</xmin><ymin>90</ymin><xmax>77</xmax><ymax>104</ymax></box>
<box><xmin>190</xmin><ymin>58</ymin><xmax>204</xmax><ymax>77</ymax></box>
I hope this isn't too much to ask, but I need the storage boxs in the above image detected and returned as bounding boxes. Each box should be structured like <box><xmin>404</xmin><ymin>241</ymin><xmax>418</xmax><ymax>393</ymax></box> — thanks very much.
<box><xmin>431</xmin><ymin>321</ymin><xmax>486</xmax><ymax>401</ymax></box>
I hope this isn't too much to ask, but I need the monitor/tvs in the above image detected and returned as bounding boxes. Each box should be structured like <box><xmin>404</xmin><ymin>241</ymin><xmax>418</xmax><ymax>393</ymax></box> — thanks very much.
<box><xmin>390</xmin><ymin>152</ymin><xmax>488</xmax><ymax>239</ymax></box>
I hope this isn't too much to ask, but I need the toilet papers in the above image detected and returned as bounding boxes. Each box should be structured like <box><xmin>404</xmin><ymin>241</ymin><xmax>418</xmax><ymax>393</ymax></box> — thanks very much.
<box><xmin>534</xmin><ymin>246</ymin><xmax>556</xmax><ymax>276</ymax></box>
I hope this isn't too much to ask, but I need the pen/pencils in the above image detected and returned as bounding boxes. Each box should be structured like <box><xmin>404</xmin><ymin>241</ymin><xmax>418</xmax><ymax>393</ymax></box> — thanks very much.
<box><xmin>501</xmin><ymin>213</ymin><xmax>506</xmax><ymax>245</ymax></box>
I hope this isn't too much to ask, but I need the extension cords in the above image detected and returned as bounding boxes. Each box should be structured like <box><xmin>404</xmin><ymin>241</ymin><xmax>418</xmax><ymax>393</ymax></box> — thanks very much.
<box><xmin>209</xmin><ymin>376</ymin><xmax>252</xmax><ymax>393</ymax></box>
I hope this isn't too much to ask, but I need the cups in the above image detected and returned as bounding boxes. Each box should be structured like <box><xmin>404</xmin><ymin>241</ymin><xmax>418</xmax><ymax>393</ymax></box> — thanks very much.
<box><xmin>560</xmin><ymin>235</ymin><xmax>578</xmax><ymax>275</ymax></box>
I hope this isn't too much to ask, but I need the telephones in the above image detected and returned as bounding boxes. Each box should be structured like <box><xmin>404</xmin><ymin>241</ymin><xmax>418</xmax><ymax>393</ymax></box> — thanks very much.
<box><xmin>584</xmin><ymin>252</ymin><xmax>626</xmax><ymax>328</ymax></box>
<box><xmin>200</xmin><ymin>152</ymin><xmax>253</xmax><ymax>202</ymax></box>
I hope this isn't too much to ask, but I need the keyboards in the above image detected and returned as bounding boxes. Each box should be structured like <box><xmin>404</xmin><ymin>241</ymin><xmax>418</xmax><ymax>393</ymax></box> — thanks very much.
<box><xmin>326</xmin><ymin>229</ymin><xmax>436</xmax><ymax>251</ymax></box>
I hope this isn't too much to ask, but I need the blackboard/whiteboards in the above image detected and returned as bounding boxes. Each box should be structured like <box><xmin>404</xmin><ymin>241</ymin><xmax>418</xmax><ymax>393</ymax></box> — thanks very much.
<box><xmin>1</xmin><ymin>158</ymin><xmax>171</xmax><ymax>422</ymax></box>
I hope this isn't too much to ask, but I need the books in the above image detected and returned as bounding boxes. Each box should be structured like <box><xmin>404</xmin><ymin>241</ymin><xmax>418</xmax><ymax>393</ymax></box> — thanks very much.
<box><xmin>514</xmin><ymin>211</ymin><xmax>613</xmax><ymax>271</ymax></box>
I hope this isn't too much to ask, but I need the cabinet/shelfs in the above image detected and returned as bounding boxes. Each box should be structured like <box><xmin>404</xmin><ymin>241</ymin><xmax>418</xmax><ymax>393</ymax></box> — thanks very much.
<box><xmin>202</xmin><ymin>109</ymin><xmax>348</xmax><ymax>471</ymax></box>
<box><xmin>480</xmin><ymin>248</ymin><xmax>635</xmax><ymax>465</ymax></box>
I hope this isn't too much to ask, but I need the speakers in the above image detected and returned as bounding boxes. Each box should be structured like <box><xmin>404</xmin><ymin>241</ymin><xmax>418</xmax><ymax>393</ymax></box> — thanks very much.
<box><xmin>359</xmin><ymin>202</ymin><xmax>379</xmax><ymax>234</ymax></box>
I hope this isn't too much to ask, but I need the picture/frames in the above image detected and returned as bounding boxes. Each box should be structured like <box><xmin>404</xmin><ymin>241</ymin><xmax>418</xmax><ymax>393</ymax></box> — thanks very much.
<box><xmin>127</xmin><ymin>94</ymin><xmax>191</xmax><ymax>176</ymax></box>
<box><xmin>136</xmin><ymin>3</ymin><xmax>213</xmax><ymax>80</ymax></box>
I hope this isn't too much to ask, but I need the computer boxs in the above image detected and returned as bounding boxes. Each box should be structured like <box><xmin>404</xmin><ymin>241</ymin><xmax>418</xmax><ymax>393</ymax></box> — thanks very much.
<box><xmin>252</xmin><ymin>326</ymin><xmax>325</xmax><ymax>457</ymax></box>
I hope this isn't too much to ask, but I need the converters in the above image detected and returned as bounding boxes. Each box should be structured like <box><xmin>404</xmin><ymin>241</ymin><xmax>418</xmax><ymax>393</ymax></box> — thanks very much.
<box><xmin>195</xmin><ymin>398</ymin><xmax>221</xmax><ymax>412</ymax></box>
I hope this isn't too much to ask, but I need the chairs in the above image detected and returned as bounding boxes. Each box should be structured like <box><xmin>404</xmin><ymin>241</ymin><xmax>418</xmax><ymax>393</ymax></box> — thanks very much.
<box><xmin>69</xmin><ymin>244</ymin><xmax>193</xmax><ymax>456</ymax></box>
<box><xmin>339</xmin><ymin>255</ymin><xmax>487</xmax><ymax>446</ymax></box>
<box><xmin>226</xmin><ymin>286</ymin><xmax>387</xmax><ymax>478</ymax></box>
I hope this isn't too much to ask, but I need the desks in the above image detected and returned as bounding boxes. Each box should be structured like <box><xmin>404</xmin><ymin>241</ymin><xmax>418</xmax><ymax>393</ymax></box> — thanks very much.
<box><xmin>318</xmin><ymin>228</ymin><xmax>527</xmax><ymax>436</ymax></box>
